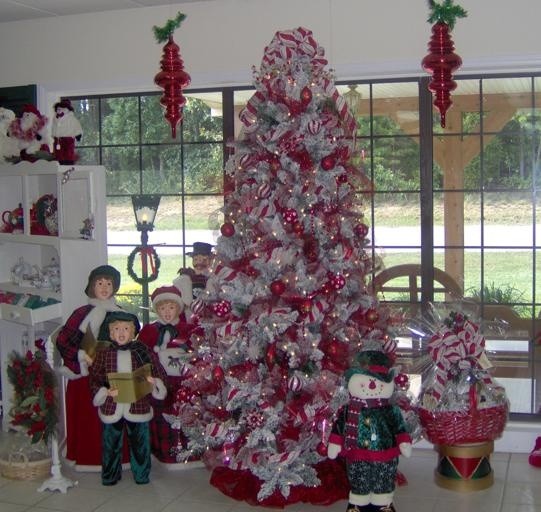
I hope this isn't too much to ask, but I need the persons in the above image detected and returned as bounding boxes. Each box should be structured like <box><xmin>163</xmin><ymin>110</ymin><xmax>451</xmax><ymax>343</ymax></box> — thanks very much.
<box><xmin>137</xmin><ymin>284</ymin><xmax>200</xmax><ymax>381</ymax></box>
<box><xmin>87</xmin><ymin>309</ymin><xmax>170</xmax><ymax>485</ymax></box>
<box><xmin>9</xmin><ymin>101</ymin><xmax>49</xmax><ymax>165</ymax></box>
<box><xmin>0</xmin><ymin>107</ymin><xmax>16</xmax><ymax>162</ymax></box>
<box><xmin>175</xmin><ymin>241</ymin><xmax>217</xmax><ymax>325</ymax></box>
<box><xmin>50</xmin><ymin>98</ymin><xmax>83</xmax><ymax>166</ymax></box>
<box><xmin>327</xmin><ymin>347</ymin><xmax>415</xmax><ymax>512</ymax></box>
<box><xmin>57</xmin><ymin>263</ymin><xmax>127</xmax><ymax>377</ymax></box>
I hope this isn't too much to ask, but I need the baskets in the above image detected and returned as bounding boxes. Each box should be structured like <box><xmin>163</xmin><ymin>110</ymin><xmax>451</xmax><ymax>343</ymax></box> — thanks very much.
<box><xmin>414</xmin><ymin>385</ymin><xmax>507</xmax><ymax>444</ymax></box>
<box><xmin>0</xmin><ymin>452</ymin><xmax>52</xmax><ymax>481</ymax></box>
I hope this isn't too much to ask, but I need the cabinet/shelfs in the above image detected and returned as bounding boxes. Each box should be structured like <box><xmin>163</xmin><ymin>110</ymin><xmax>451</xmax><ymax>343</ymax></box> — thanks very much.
<box><xmin>0</xmin><ymin>162</ymin><xmax>110</xmax><ymax>457</ymax></box>
